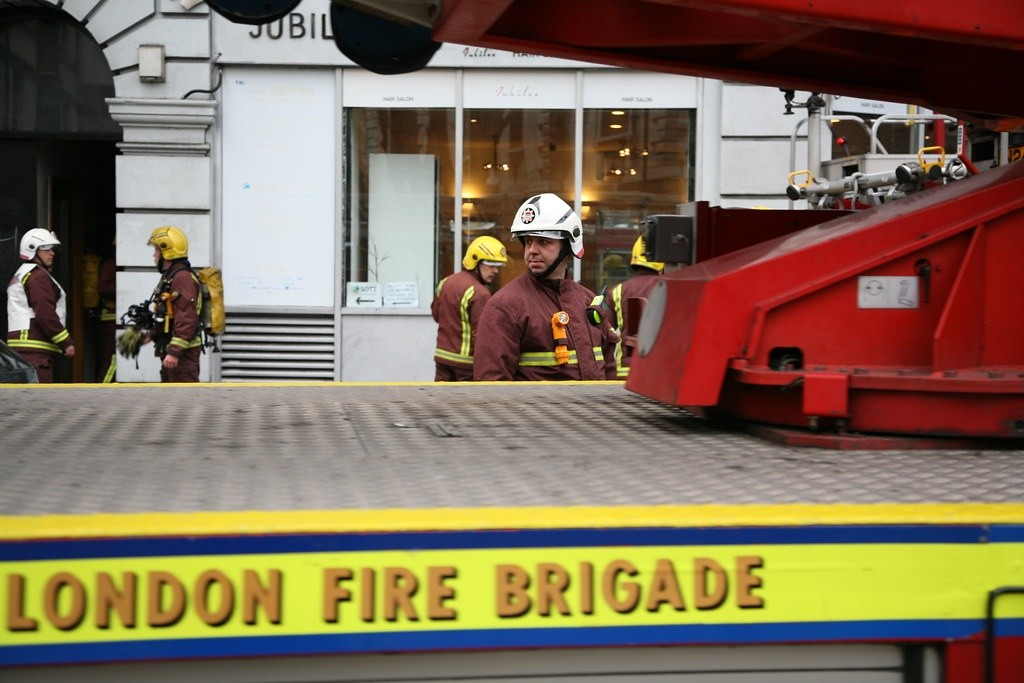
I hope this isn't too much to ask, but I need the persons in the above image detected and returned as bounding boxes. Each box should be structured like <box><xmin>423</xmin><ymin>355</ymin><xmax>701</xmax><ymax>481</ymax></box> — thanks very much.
<box><xmin>609</xmin><ymin>232</ymin><xmax>668</xmax><ymax>382</ymax></box>
<box><xmin>95</xmin><ymin>234</ymin><xmax>117</xmax><ymax>385</ymax></box>
<box><xmin>148</xmin><ymin>226</ymin><xmax>203</xmax><ymax>383</ymax></box>
<box><xmin>7</xmin><ymin>229</ymin><xmax>75</xmax><ymax>385</ymax></box>
<box><xmin>471</xmin><ymin>192</ymin><xmax>616</xmax><ymax>382</ymax></box>
<box><xmin>430</xmin><ymin>235</ymin><xmax>508</xmax><ymax>382</ymax></box>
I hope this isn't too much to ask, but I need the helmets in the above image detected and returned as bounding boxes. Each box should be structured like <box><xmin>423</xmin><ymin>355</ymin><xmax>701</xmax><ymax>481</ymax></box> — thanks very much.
<box><xmin>631</xmin><ymin>234</ymin><xmax>665</xmax><ymax>271</ymax></box>
<box><xmin>463</xmin><ymin>236</ymin><xmax>508</xmax><ymax>270</ymax></box>
<box><xmin>148</xmin><ymin>225</ymin><xmax>189</xmax><ymax>259</ymax></box>
<box><xmin>603</xmin><ymin>255</ymin><xmax>627</xmax><ymax>268</ymax></box>
<box><xmin>19</xmin><ymin>228</ymin><xmax>63</xmax><ymax>260</ymax></box>
<box><xmin>511</xmin><ymin>193</ymin><xmax>586</xmax><ymax>259</ymax></box>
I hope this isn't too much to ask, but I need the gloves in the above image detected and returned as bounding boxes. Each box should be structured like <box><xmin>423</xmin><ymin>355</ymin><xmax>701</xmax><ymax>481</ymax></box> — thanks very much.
<box><xmin>117</xmin><ymin>327</ymin><xmax>145</xmax><ymax>359</ymax></box>
